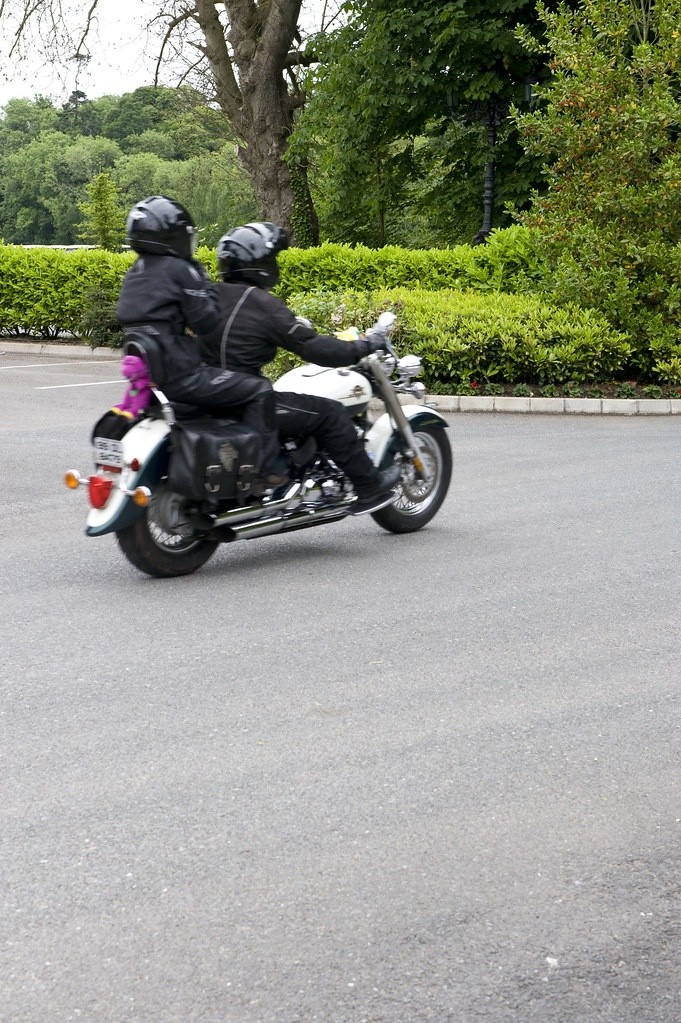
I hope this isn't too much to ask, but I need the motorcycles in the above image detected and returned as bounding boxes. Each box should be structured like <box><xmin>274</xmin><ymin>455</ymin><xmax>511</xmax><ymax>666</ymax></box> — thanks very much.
<box><xmin>64</xmin><ymin>307</ymin><xmax>453</xmax><ymax>578</ymax></box>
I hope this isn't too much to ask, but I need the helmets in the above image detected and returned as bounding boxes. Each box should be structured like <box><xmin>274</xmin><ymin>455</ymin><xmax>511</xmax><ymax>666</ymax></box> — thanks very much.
<box><xmin>125</xmin><ymin>193</ymin><xmax>198</xmax><ymax>263</ymax></box>
<box><xmin>215</xmin><ymin>220</ymin><xmax>291</xmax><ymax>289</ymax></box>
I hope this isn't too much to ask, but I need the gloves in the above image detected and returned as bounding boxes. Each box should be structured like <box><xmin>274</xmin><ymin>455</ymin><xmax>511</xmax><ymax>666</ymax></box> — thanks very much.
<box><xmin>365</xmin><ymin>330</ymin><xmax>389</xmax><ymax>352</ymax></box>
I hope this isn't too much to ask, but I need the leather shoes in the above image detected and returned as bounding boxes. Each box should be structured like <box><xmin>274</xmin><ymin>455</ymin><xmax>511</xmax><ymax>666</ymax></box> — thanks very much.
<box><xmin>356</xmin><ymin>462</ymin><xmax>401</xmax><ymax>505</ymax></box>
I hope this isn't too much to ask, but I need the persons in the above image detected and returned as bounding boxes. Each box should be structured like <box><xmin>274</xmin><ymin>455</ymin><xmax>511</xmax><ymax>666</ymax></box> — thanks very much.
<box><xmin>115</xmin><ymin>196</ymin><xmax>288</xmax><ymax>487</ymax></box>
<box><xmin>195</xmin><ymin>222</ymin><xmax>403</xmax><ymax>505</ymax></box>
